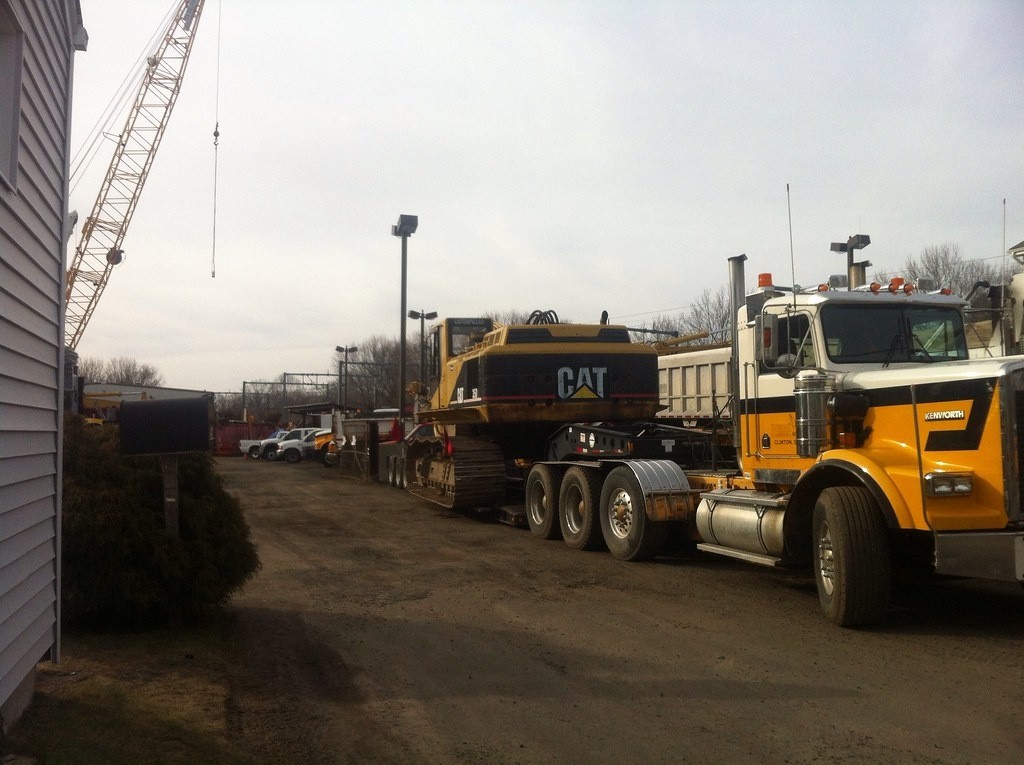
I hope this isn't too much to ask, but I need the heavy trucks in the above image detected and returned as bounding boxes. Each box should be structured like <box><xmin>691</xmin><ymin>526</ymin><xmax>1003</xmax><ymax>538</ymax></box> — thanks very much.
<box><xmin>386</xmin><ymin>253</ymin><xmax>1023</xmax><ymax>630</ymax></box>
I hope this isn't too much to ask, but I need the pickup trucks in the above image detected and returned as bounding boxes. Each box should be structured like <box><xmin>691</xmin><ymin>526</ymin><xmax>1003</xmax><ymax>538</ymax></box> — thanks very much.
<box><xmin>239</xmin><ymin>431</ymin><xmax>288</xmax><ymax>458</ymax></box>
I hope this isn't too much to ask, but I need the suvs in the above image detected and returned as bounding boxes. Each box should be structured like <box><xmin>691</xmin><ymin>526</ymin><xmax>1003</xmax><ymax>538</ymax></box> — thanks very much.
<box><xmin>277</xmin><ymin>428</ymin><xmax>322</xmax><ymax>463</ymax></box>
<box><xmin>260</xmin><ymin>427</ymin><xmax>316</xmax><ymax>461</ymax></box>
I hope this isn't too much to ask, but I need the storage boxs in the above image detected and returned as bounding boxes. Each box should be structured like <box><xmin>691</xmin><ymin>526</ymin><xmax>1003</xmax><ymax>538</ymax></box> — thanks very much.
<box><xmin>118</xmin><ymin>396</ymin><xmax>210</xmax><ymax>456</ymax></box>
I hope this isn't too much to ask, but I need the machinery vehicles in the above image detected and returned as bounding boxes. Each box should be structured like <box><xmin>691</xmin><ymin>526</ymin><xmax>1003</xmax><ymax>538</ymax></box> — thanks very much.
<box><xmin>64</xmin><ymin>0</ymin><xmax>204</xmax><ymax>432</ymax></box>
<box><xmin>401</xmin><ymin>316</ymin><xmax>713</xmax><ymax>508</ymax></box>
